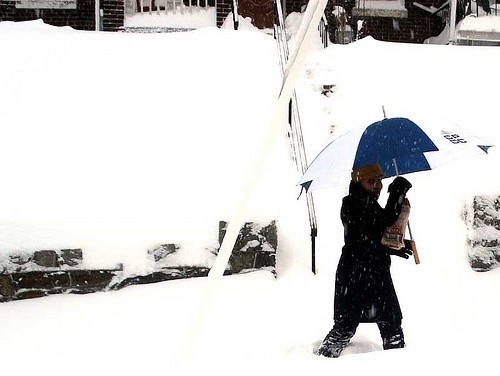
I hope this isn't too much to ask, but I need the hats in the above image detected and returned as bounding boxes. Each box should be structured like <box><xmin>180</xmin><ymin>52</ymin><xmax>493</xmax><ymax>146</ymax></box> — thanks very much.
<box><xmin>351</xmin><ymin>163</ymin><xmax>384</xmax><ymax>183</ymax></box>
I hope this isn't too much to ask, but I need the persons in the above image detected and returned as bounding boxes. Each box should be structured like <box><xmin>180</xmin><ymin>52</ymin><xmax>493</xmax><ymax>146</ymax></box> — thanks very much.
<box><xmin>313</xmin><ymin>162</ymin><xmax>405</xmax><ymax>360</ymax></box>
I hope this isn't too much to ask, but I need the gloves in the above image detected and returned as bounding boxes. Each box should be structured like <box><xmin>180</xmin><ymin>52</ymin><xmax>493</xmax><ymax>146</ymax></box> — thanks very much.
<box><xmin>388</xmin><ymin>240</ymin><xmax>413</xmax><ymax>259</ymax></box>
<box><xmin>387</xmin><ymin>177</ymin><xmax>412</xmax><ymax>197</ymax></box>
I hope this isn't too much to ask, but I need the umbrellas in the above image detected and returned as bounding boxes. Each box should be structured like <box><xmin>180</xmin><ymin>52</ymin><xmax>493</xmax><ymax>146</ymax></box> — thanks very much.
<box><xmin>351</xmin><ymin>105</ymin><xmax>440</xmax><ymax>262</ymax></box>
